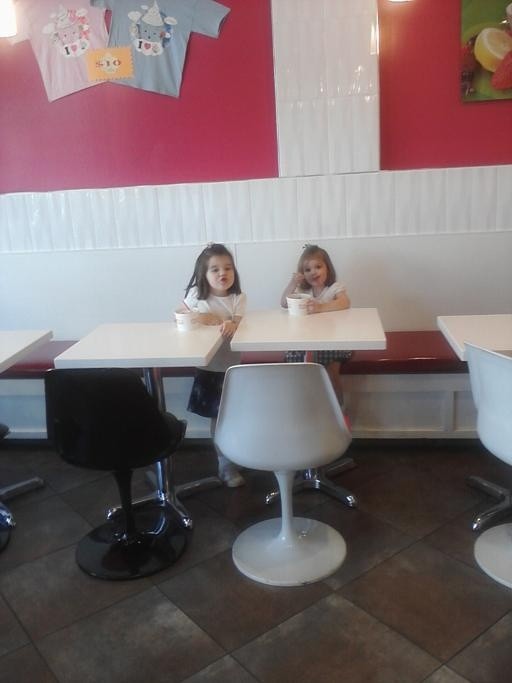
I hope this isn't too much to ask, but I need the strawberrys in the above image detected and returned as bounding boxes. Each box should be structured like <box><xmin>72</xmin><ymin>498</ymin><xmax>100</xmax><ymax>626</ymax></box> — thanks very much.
<box><xmin>491</xmin><ymin>50</ymin><xmax>512</xmax><ymax>89</ymax></box>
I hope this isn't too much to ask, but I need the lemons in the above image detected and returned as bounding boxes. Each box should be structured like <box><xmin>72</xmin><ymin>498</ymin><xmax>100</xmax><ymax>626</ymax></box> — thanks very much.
<box><xmin>474</xmin><ymin>28</ymin><xmax>512</xmax><ymax>71</ymax></box>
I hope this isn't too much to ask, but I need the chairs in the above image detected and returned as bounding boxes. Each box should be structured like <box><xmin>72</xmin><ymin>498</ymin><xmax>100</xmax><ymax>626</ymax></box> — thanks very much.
<box><xmin>209</xmin><ymin>362</ymin><xmax>355</xmax><ymax>589</ymax></box>
<box><xmin>465</xmin><ymin>340</ymin><xmax>512</xmax><ymax>591</ymax></box>
<box><xmin>44</xmin><ymin>366</ymin><xmax>188</xmax><ymax>583</ymax></box>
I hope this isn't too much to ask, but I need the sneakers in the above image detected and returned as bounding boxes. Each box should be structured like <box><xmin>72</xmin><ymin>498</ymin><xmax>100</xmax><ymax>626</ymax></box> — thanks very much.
<box><xmin>219</xmin><ymin>464</ymin><xmax>246</xmax><ymax>487</ymax></box>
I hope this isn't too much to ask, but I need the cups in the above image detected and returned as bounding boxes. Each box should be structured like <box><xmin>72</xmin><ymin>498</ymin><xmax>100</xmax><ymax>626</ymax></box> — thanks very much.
<box><xmin>175</xmin><ymin>306</ymin><xmax>200</xmax><ymax>332</ymax></box>
<box><xmin>285</xmin><ymin>292</ymin><xmax>312</xmax><ymax>314</ymax></box>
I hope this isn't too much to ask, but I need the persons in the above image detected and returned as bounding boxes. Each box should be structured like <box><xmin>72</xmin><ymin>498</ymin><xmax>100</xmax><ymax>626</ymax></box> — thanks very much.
<box><xmin>181</xmin><ymin>240</ymin><xmax>248</xmax><ymax>490</ymax></box>
<box><xmin>280</xmin><ymin>242</ymin><xmax>354</xmax><ymax>437</ymax></box>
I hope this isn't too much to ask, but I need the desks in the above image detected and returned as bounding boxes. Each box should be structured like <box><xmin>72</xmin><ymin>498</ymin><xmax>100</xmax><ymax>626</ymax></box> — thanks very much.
<box><xmin>432</xmin><ymin>313</ymin><xmax>512</xmax><ymax>538</ymax></box>
<box><xmin>0</xmin><ymin>326</ymin><xmax>53</xmax><ymax>527</ymax></box>
<box><xmin>228</xmin><ymin>305</ymin><xmax>389</xmax><ymax>514</ymax></box>
<box><xmin>52</xmin><ymin>320</ymin><xmax>228</xmax><ymax>531</ymax></box>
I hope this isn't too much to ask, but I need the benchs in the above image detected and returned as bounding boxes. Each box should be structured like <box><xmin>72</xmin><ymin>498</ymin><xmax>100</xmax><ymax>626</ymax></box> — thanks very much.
<box><xmin>0</xmin><ymin>329</ymin><xmax>475</xmax><ymax>401</ymax></box>
